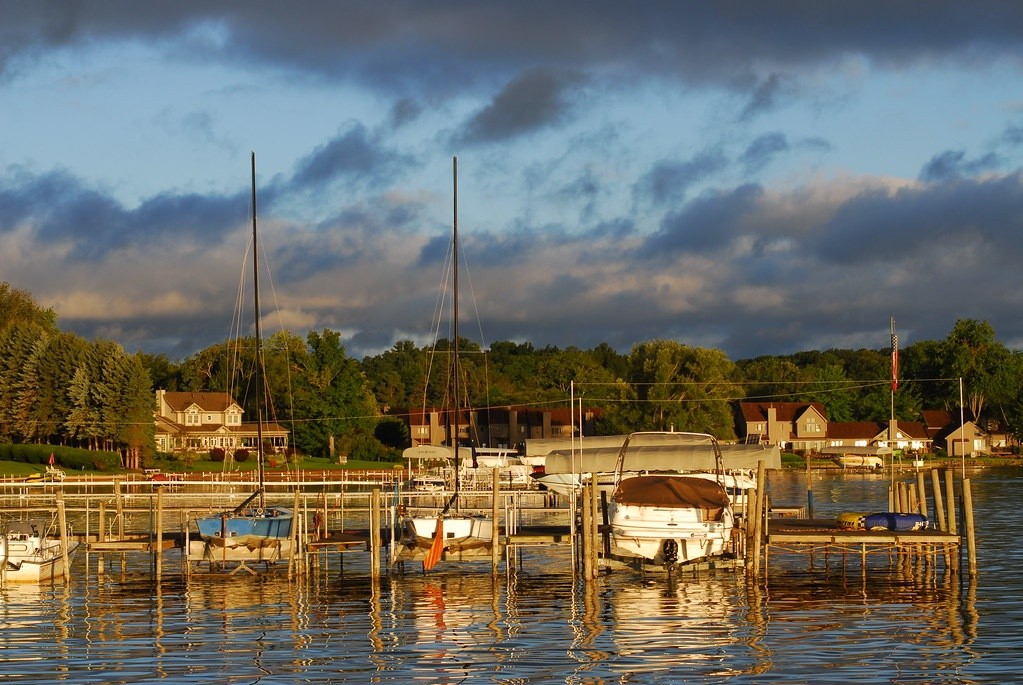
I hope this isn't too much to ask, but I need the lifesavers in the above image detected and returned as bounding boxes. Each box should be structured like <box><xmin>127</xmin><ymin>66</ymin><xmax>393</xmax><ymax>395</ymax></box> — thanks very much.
<box><xmin>257</xmin><ymin>508</ymin><xmax>263</xmax><ymax>515</ymax></box>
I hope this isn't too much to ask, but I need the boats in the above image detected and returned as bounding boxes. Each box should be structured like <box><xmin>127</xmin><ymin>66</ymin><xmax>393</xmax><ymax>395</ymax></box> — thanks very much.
<box><xmin>831</xmin><ymin>454</ymin><xmax>882</xmax><ymax>469</ymax></box>
<box><xmin>24</xmin><ymin>467</ymin><xmax>66</xmax><ymax>483</ymax></box>
<box><xmin>0</xmin><ymin>530</ymin><xmax>81</xmax><ymax>583</ymax></box>
<box><xmin>607</xmin><ymin>423</ymin><xmax>734</xmax><ymax>564</ymax></box>
<box><xmin>401</xmin><ymin>443</ymin><xmax>546</xmax><ymax>490</ymax></box>
<box><xmin>528</xmin><ymin>443</ymin><xmax>782</xmax><ymax>520</ymax></box>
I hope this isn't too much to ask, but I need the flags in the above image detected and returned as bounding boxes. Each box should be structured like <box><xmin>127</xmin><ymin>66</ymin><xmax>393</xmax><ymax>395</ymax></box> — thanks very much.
<box><xmin>49</xmin><ymin>453</ymin><xmax>54</xmax><ymax>463</ymax></box>
<box><xmin>423</xmin><ymin>521</ymin><xmax>443</xmax><ymax>571</ymax></box>
<box><xmin>891</xmin><ymin>320</ymin><xmax>899</xmax><ymax>392</ymax></box>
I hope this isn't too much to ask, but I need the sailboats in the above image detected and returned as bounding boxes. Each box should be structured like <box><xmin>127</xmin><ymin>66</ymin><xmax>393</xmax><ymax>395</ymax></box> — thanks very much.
<box><xmin>195</xmin><ymin>151</ymin><xmax>293</xmax><ymax>544</ymax></box>
<box><xmin>400</xmin><ymin>155</ymin><xmax>492</xmax><ymax>544</ymax></box>
